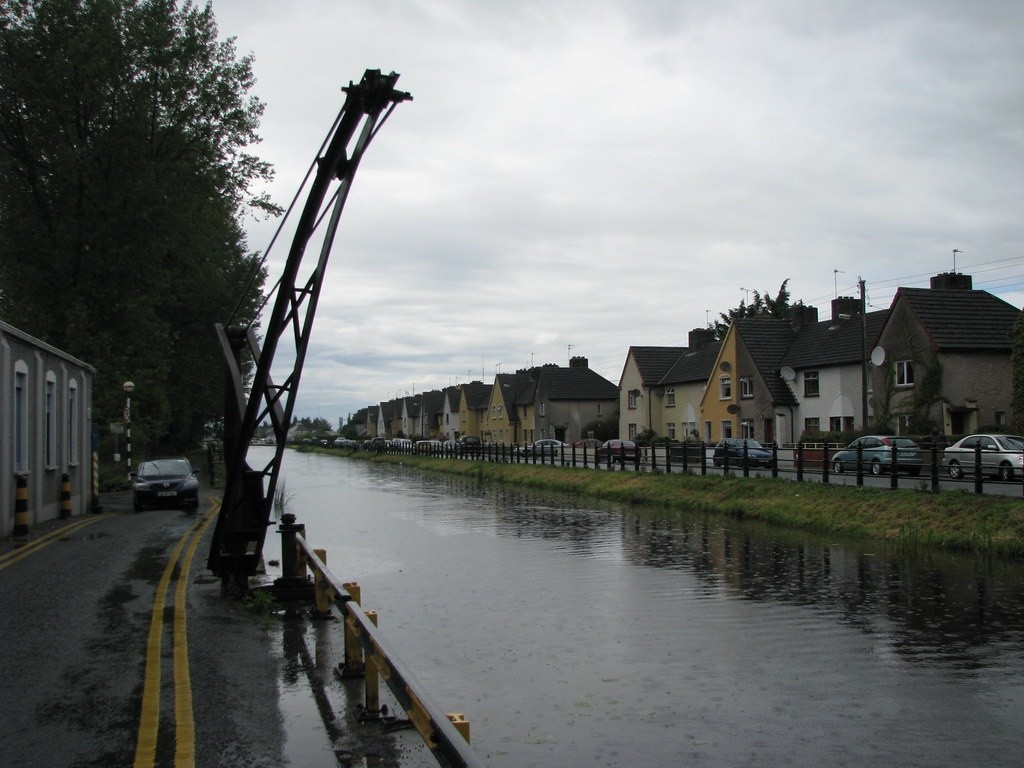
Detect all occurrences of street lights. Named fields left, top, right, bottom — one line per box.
left=836, top=312, right=871, bottom=436
left=369, top=412, right=378, bottom=437
left=413, top=397, right=424, bottom=440
left=121, top=380, right=137, bottom=492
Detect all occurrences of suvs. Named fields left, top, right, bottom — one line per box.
left=713, top=435, right=773, bottom=469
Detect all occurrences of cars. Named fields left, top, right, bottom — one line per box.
left=941, top=433, right=1024, bottom=483
left=830, top=435, right=924, bottom=477
left=302, top=432, right=482, bottom=458
left=513, top=438, right=571, bottom=458
left=575, top=438, right=604, bottom=449
left=129, top=457, right=202, bottom=512
left=594, top=439, right=642, bottom=464
